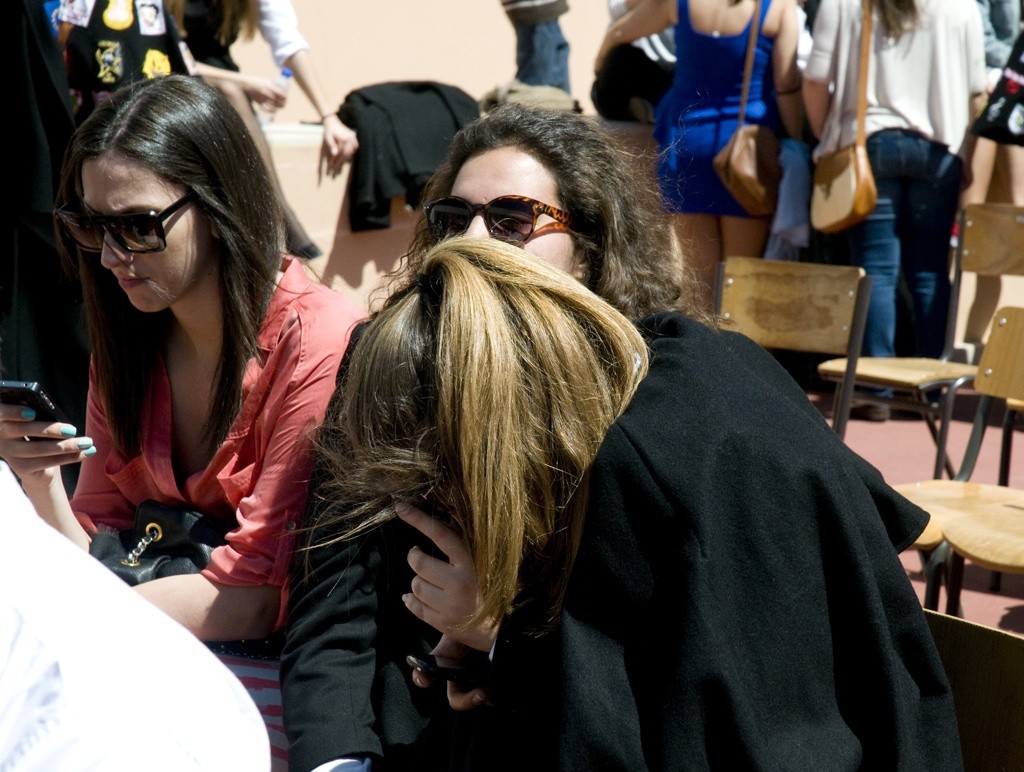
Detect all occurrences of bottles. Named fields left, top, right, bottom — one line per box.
left=258, top=67, right=293, bottom=123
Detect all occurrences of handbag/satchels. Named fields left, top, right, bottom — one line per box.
left=810, top=145, right=878, bottom=234
left=713, top=126, right=780, bottom=215
left=77, top=496, right=282, bottom=659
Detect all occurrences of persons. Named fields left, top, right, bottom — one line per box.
left=0, top=0, right=1024, bottom=772
left=319, top=234, right=968, bottom=772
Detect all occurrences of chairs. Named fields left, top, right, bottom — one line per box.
left=598, top=204, right=1024, bottom=772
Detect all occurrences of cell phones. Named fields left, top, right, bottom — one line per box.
left=0, top=380, right=68, bottom=441
left=407, top=656, right=494, bottom=691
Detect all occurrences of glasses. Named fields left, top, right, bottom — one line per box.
left=422, top=197, right=575, bottom=249
left=55, top=189, right=196, bottom=253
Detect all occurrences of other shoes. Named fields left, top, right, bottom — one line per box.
left=887, top=391, right=944, bottom=418
left=813, top=398, right=890, bottom=422
